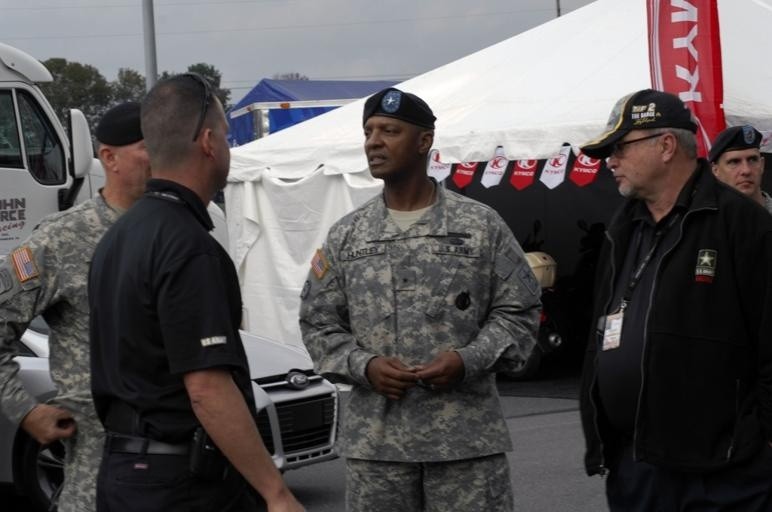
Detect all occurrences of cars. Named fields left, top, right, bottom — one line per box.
left=1, top=309, right=341, bottom=508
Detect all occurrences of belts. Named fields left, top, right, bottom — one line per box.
left=104, top=432, right=193, bottom=454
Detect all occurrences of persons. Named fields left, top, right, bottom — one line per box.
left=0, top=102, right=150, bottom=512
left=581, top=89, right=771, bottom=512
left=88, top=75, right=308, bottom=512
left=298, top=89, right=542, bottom=512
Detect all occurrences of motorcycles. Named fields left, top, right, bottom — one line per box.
left=520, top=219, right=602, bottom=253
left=496, top=252, right=564, bottom=379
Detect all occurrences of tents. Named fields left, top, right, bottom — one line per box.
left=221, top=1, right=771, bottom=352
left=228, top=77, right=402, bottom=147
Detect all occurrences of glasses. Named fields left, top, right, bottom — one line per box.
left=182, top=73, right=212, bottom=141
left=612, top=133, right=663, bottom=157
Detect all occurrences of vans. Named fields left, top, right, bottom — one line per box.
left=0, top=43, right=108, bottom=266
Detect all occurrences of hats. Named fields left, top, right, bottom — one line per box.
left=578, top=89, right=698, bottom=160
left=364, top=88, right=437, bottom=130
left=95, top=102, right=144, bottom=146
left=709, top=126, right=763, bottom=163
